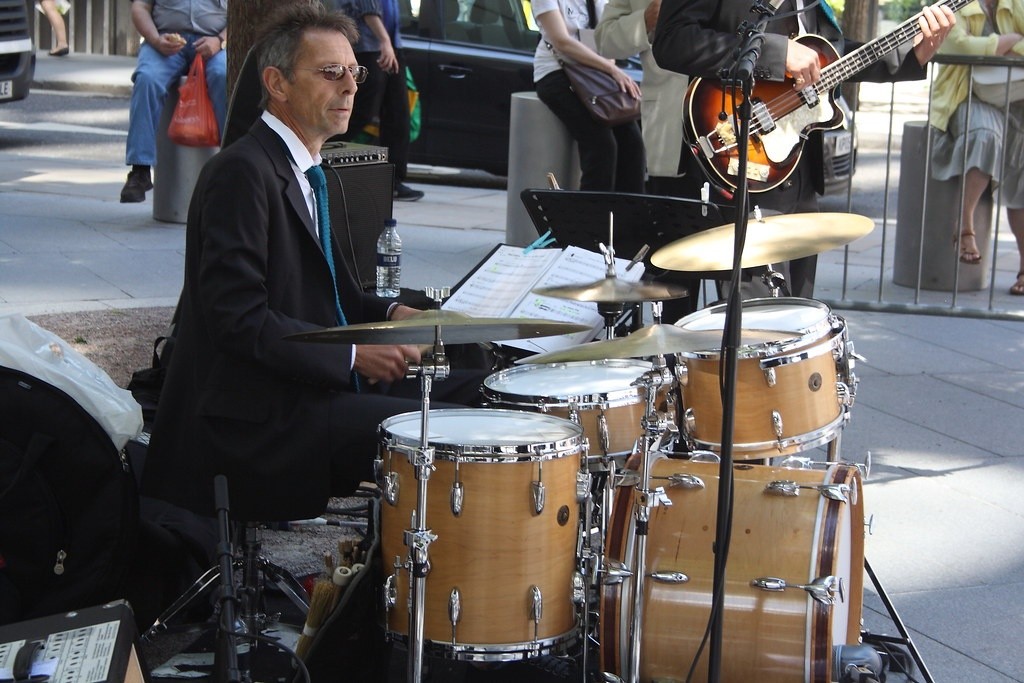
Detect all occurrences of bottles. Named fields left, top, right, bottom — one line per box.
left=216, top=600, right=251, bottom=678
left=377, top=219, right=402, bottom=298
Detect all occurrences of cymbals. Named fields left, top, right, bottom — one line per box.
left=529, top=278, right=690, bottom=303
left=281, top=309, right=595, bottom=345
left=513, top=323, right=807, bottom=364
left=650, top=212, right=875, bottom=272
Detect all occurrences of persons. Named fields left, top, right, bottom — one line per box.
left=119, top=0, right=228, bottom=206
left=35, top=0, right=71, bottom=56
left=928, top=0, right=1024, bottom=296
left=528, top=1, right=955, bottom=469
left=332, top=0, right=423, bottom=201
left=151, top=6, right=489, bottom=683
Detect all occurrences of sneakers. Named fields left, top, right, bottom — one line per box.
left=120, top=170, right=154, bottom=203
left=393, top=183, right=424, bottom=202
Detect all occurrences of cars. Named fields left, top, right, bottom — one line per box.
left=354, top=1, right=858, bottom=199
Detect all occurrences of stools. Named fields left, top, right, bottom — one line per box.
left=139, top=489, right=312, bottom=683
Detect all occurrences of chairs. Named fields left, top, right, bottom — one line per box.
left=444, top=0, right=471, bottom=43
left=470, top=0, right=513, bottom=49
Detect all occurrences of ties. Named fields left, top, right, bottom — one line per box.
left=305, top=165, right=361, bottom=393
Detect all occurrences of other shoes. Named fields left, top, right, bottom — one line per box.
left=48, top=47, right=68, bottom=57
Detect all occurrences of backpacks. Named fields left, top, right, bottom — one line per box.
left=0, top=326, right=178, bottom=629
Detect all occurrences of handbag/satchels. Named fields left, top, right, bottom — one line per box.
left=559, top=57, right=642, bottom=126
left=167, top=53, right=221, bottom=147
left=970, top=58, right=1024, bottom=107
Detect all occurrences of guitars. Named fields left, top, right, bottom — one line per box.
left=681, top=0, right=975, bottom=193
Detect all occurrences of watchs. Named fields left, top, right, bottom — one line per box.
left=215, top=35, right=226, bottom=50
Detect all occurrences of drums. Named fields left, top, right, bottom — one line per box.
left=379, top=408, right=583, bottom=663
left=674, top=296, right=847, bottom=460
left=478, top=359, right=671, bottom=464
left=599, top=451, right=865, bottom=683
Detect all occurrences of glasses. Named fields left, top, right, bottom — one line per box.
left=288, top=65, right=368, bottom=84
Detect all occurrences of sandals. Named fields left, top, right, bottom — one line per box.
left=952, top=230, right=984, bottom=265
left=1009, top=271, right=1024, bottom=295
